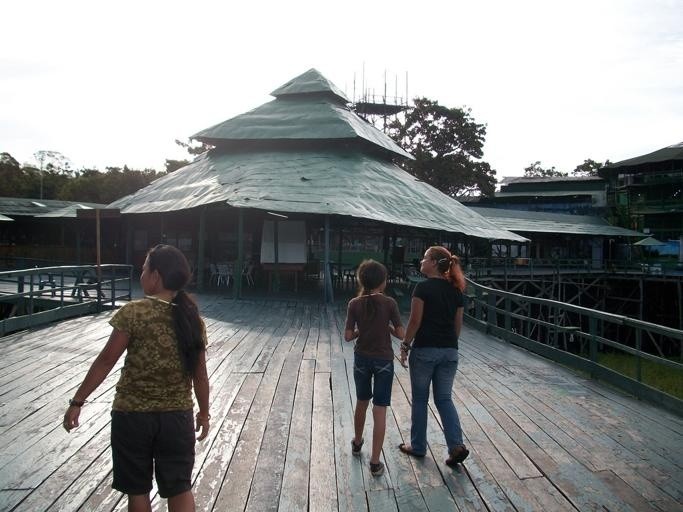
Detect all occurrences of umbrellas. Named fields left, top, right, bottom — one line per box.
left=631, top=237, right=668, bottom=258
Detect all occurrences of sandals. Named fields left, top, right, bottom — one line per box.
left=399, top=443, right=426, bottom=457
left=446, top=444, right=469, bottom=465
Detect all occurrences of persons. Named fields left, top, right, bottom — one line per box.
left=60, top=244, right=212, bottom=511
left=341, top=258, right=404, bottom=477
left=397, top=245, right=469, bottom=466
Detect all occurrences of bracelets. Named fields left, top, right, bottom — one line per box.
left=68, top=397, right=87, bottom=407
left=195, top=412, right=210, bottom=422
left=399, top=341, right=411, bottom=356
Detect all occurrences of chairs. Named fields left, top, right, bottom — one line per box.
left=384, top=262, right=402, bottom=284
left=330, top=263, right=361, bottom=287
left=210, top=259, right=255, bottom=286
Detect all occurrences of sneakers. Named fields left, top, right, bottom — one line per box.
left=351, top=438, right=364, bottom=455
left=370, top=462, right=384, bottom=476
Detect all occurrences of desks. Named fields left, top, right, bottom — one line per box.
left=404, top=262, right=428, bottom=289
